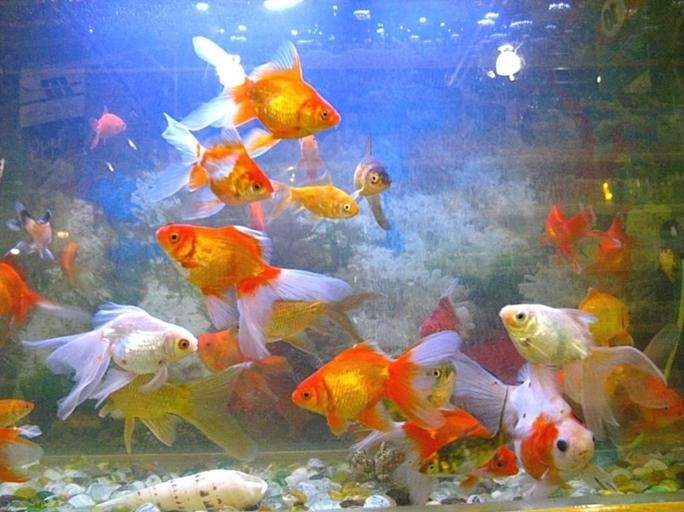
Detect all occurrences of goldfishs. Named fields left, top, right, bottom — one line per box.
left=0, top=35, right=684, bottom=511
